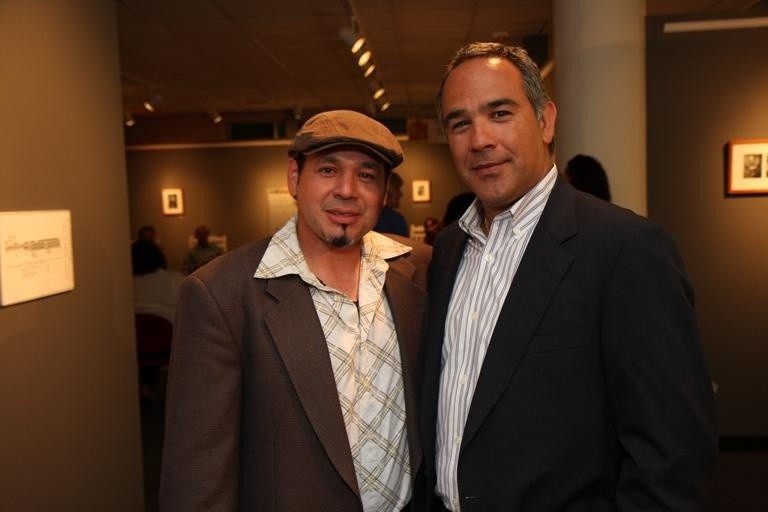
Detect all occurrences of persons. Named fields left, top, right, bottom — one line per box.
left=152, top=109, right=434, bottom=511
left=179, top=224, right=225, bottom=273
left=422, top=217, right=443, bottom=245
left=373, top=170, right=408, bottom=238
left=414, top=42, right=717, bottom=511
left=131, top=225, right=168, bottom=277
left=564, top=154, right=611, bottom=202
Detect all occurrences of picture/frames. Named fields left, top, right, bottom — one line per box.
left=725, top=135, right=768, bottom=197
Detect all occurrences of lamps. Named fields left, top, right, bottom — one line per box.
left=337, top=22, right=390, bottom=116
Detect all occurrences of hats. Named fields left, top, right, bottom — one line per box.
left=288, top=109, right=405, bottom=171
